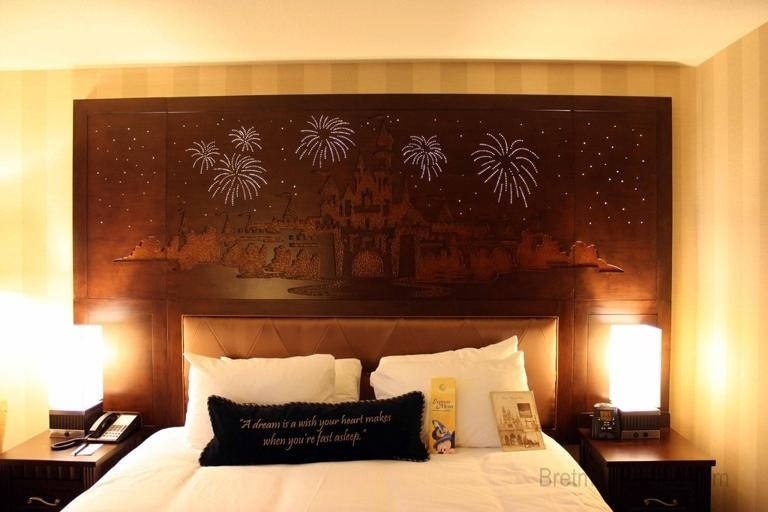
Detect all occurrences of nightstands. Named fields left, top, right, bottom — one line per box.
left=2, top=428, right=137, bottom=512
left=581, top=427, right=716, bottom=512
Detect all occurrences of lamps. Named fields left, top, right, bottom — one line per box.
left=48, top=325, right=105, bottom=431
left=611, top=325, right=664, bottom=441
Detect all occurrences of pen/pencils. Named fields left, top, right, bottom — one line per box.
left=74, top=443, right=90, bottom=456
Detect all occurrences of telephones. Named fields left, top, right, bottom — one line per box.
left=50, top=412, right=142, bottom=451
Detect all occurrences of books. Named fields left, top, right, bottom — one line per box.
left=490, top=390, right=545, bottom=452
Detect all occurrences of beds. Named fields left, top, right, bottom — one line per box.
left=57, top=313, right=612, bottom=511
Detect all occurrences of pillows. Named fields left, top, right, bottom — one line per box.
left=178, top=334, right=532, bottom=465
